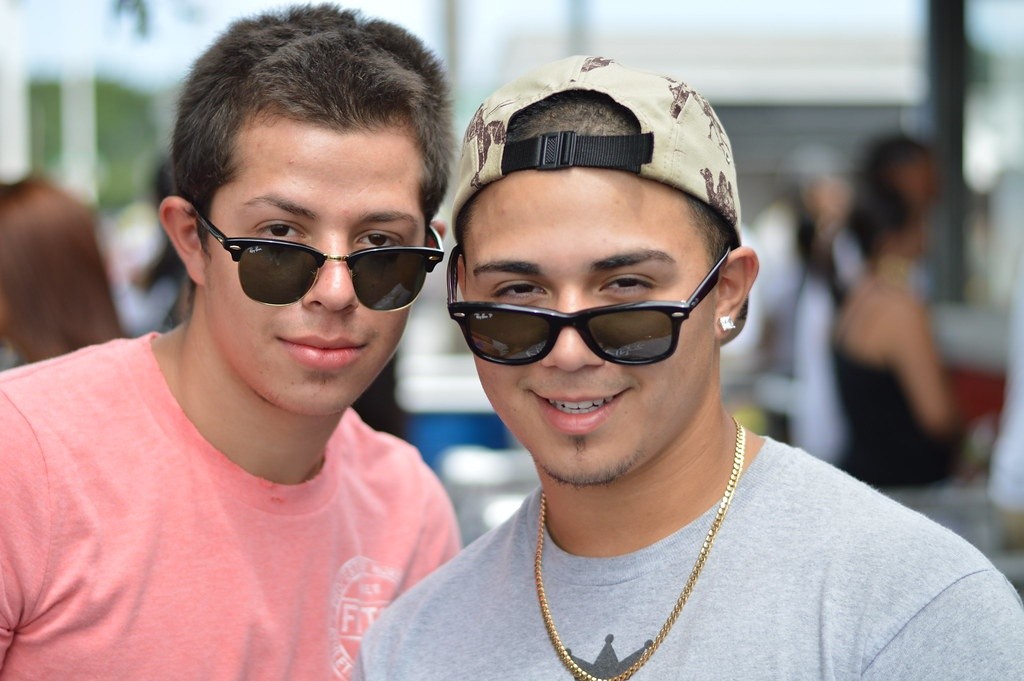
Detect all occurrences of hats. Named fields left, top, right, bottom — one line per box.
left=450, top=55, right=749, bottom=346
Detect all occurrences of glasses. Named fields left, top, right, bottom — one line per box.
left=446, top=243, right=732, bottom=364
left=193, top=209, right=444, bottom=311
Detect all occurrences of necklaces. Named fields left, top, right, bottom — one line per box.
left=534, top=416, right=746, bottom=681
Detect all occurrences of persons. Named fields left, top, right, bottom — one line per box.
left=0, top=180, right=124, bottom=364
left=0, top=3, right=461, bottom=681
left=749, top=135, right=1023, bottom=552
left=354, top=57, right=1024, bottom=681
